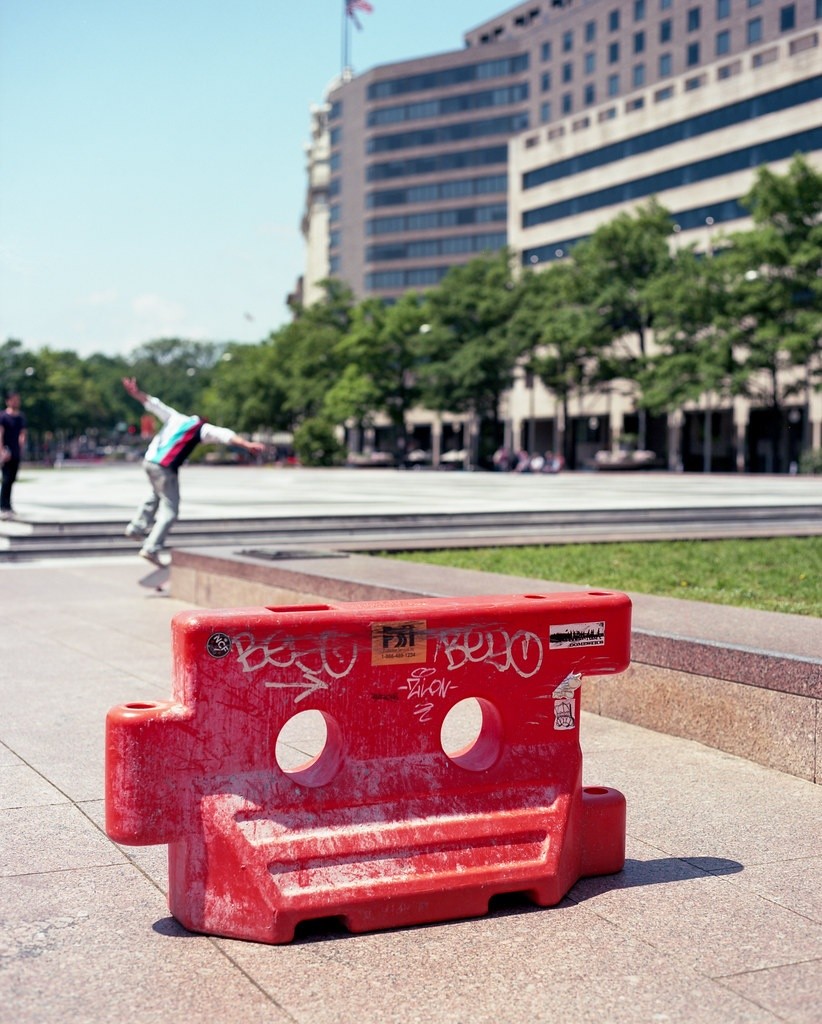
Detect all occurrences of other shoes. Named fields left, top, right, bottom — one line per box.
left=126, top=528, right=147, bottom=540
left=138, top=549, right=167, bottom=569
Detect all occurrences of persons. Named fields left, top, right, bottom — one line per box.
left=0, top=389, right=29, bottom=519
left=120, top=375, right=269, bottom=565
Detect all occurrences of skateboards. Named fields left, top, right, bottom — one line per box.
left=137, top=562, right=172, bottom=593
